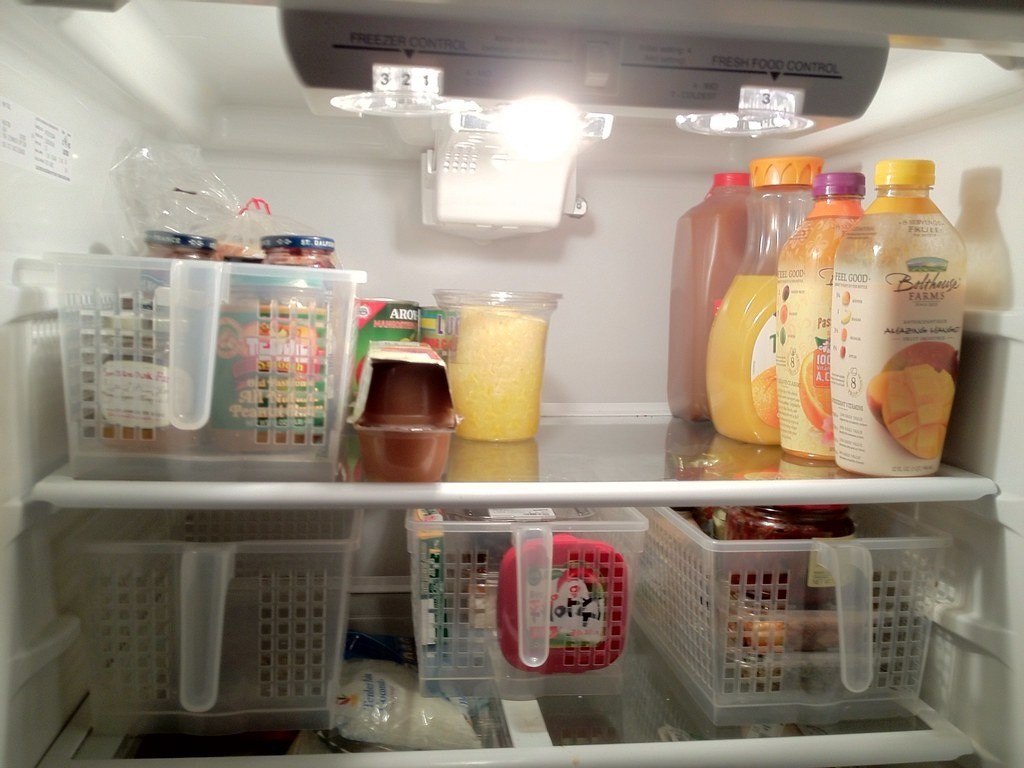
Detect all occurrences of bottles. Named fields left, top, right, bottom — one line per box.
left=829, top=158, right=969, bottom=479
left=666, top=170, right=751, bottom=422
left=775, top=172, right=867, bottom=462
left=705, top=156, right=826, bottom=446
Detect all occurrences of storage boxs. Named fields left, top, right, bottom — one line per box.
left=50, top=253, right=367, bottom=488
left=71, top=506, right=956, bottom=728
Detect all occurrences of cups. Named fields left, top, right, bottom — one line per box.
left=432, top=288, right=563, bottom=443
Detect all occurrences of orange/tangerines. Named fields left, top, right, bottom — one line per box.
left=751, top=366, right=779, bottom=428
left=798, top=352, right=832, bottom=427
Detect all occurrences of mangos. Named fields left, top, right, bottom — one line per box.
left=878, top=364, right=955, bottom=459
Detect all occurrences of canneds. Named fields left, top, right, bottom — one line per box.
left=139, top=228, right=461, bottom=440
left=694, top=504, right=859, bottom=613
